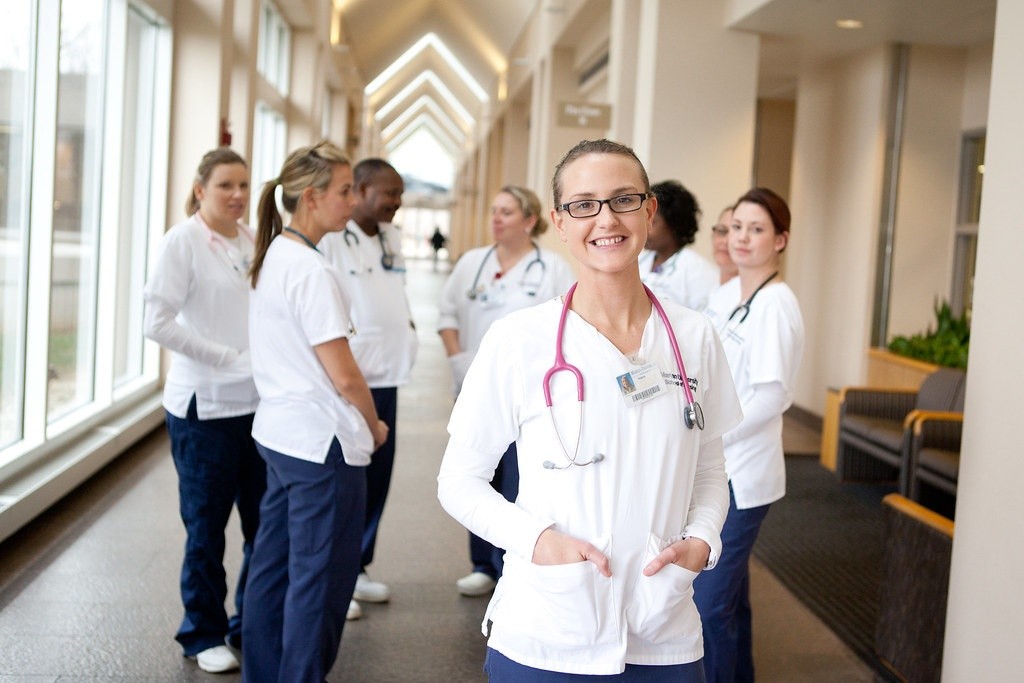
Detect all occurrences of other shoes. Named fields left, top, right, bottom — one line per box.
left=346, top=598, right=361, bottom=621
left=186, top=644, right=239, bottom=673
left=352, top=571, right=390, bottom=602
left=456, top=571, right=497, bottom=596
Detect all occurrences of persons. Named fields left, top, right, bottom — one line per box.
left=142, top=149, right=258, bottom=672
left=437, top=185, right=577, bottom=596
left=437, top=139, right=743, bottom=683
left=242, top=138, right=416, bottom=683
left=638, top=180, right=803, bottom=683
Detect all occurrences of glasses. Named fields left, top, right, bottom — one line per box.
left=558, top=192, right=649, bottom=218
left=713, top=225, right=729, bottom=236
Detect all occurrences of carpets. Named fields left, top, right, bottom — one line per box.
left=749, top=453, right=956, bottom=683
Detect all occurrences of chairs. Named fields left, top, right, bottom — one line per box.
left=817, top=365, right=968, bottom=508
left=883, top=410, right=964, bottom=549
